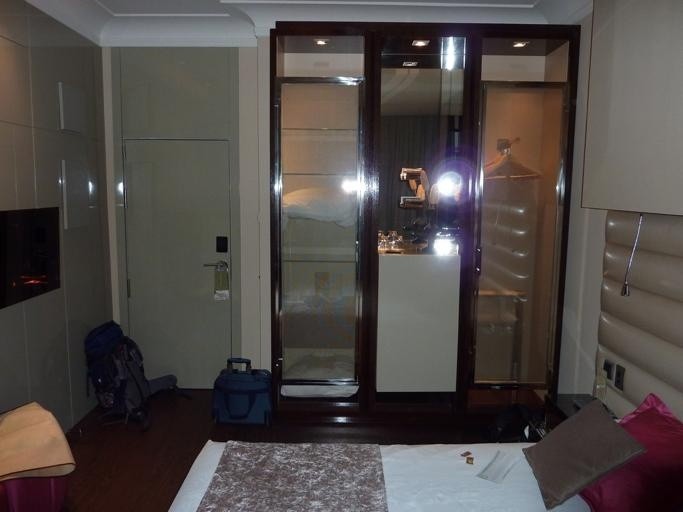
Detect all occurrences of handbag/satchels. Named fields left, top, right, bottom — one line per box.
left=213, top=359, right=272, bottom=428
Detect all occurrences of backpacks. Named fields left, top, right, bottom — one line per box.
left=84, top=320, right=175, bottom=429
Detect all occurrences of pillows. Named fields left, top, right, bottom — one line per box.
left=522, top=393, right=681, bottom=511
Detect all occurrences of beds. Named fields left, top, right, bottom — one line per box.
left=167, top=438, right=591, bottom=511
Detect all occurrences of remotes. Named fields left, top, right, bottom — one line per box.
left=573, top=399, right=587, bottom=409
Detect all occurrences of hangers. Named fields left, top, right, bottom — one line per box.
left=483, top=145, right=543, bottom=181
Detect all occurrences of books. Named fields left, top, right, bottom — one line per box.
left=572, top=398, right=593, bottom=411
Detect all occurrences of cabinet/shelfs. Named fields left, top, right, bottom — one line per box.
left=268, top=22, right=581, bottom=428
